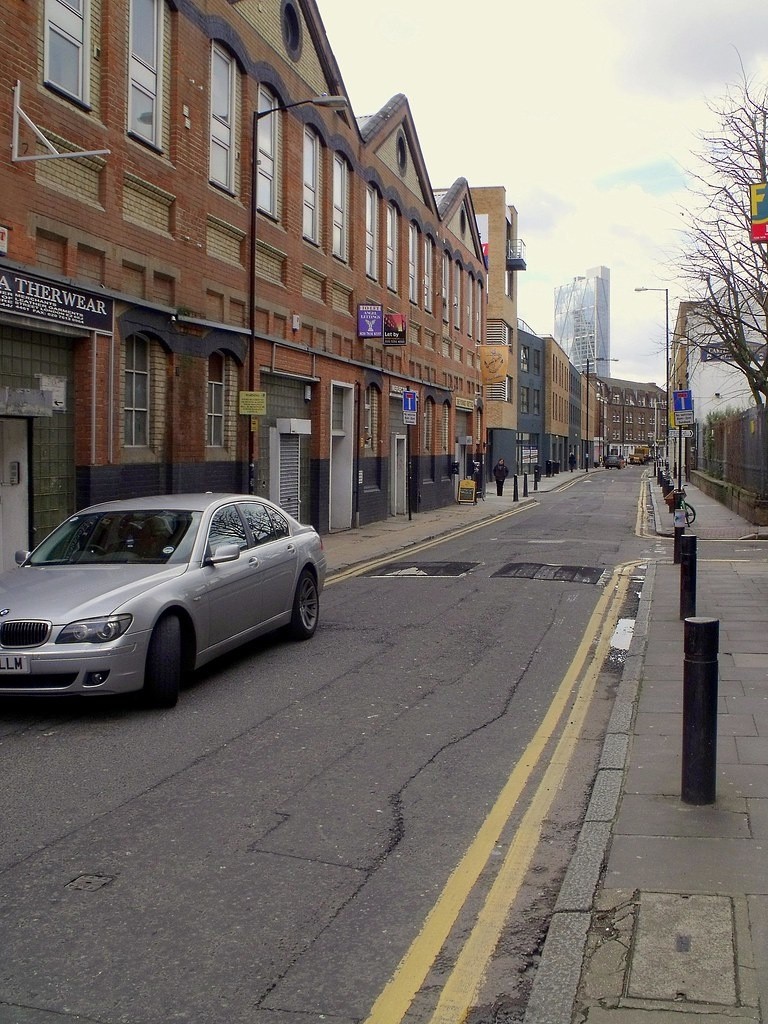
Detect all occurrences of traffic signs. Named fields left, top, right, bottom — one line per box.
left=668, top=430, right=694, bottom=437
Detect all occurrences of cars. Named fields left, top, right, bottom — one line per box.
left=0, top=492, right=326, bottom=709
left=605, top=454, right=623, bottom=470
left=633, top=459, right=641, bottom=466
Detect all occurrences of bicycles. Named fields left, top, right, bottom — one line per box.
left=674, top=483, right=699, bottom=526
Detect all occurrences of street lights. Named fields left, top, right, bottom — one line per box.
left=635, top=287, right=670, bottom=469
left=586, top=357, right=604, bottom=474
left=247, top=93, right=350, bottom=495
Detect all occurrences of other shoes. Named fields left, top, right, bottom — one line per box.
left=571, top=470, right=572, bottom=472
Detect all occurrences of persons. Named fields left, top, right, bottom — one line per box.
left=569, top=454, right=575, bottom=472
left=599, top=455, right=603, bottom=468
left=493, top=459, right=509, bottom=496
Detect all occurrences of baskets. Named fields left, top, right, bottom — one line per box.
left=665, top=488, right=687, bottom=505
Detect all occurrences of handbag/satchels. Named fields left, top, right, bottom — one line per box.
left=574, top=460, right=576, bottom=464
